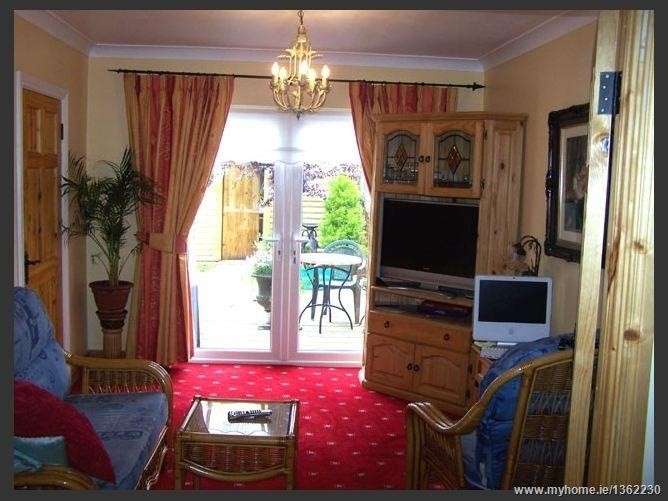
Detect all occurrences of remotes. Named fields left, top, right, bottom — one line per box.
left=229, top=409, right=273, bottom=421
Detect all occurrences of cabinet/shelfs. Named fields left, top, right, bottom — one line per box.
left=361, top=111, right=529, bottom=416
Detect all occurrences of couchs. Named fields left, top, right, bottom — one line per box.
left=14, top=287, right=173, bottom=490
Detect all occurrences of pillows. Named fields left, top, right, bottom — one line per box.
left=14, top=379, right=117, bottom=484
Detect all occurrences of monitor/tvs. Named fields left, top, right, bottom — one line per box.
left=472, top=275, right=553, bottom=345
left=377, top=194, right=481, bottom=300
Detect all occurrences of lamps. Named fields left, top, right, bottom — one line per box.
left=269, top=9, right=333, bottom=120
left=513, top=235, right=542, bottom=276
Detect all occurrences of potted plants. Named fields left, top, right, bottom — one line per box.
left=252, top=264, right=272, bottom=330
left=58, top=145, right=167, bottom=312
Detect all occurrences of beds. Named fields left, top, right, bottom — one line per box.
left=298, top=252, right=362, bottom=334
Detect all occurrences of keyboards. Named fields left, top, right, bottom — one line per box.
left=479, top=346, right=509, bottom=360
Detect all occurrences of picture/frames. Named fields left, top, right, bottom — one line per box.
left=543, top=102, right=605, bottom=269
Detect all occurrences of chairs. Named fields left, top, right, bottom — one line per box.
left=311, top=240, right=366, bottom=324
left=407, top=328, right=600, bottom=490
